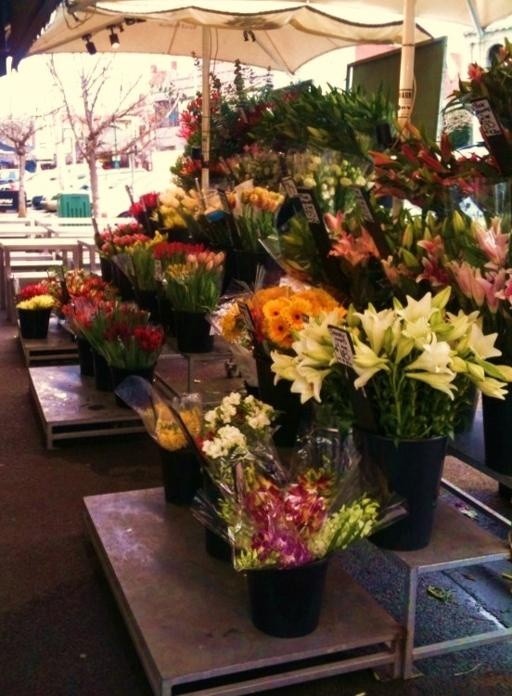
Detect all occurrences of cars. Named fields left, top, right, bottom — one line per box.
left=0, top=157, right=169, bottom=217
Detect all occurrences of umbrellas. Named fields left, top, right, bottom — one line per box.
left=22, top=7, right=433, bottom=192
left=275, top=1, right=512, bottom=133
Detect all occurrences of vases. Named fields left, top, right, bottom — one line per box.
left=248, top=555, right=330, bottom=638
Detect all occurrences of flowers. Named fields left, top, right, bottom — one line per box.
left=14, top=35, right=512, bottom=574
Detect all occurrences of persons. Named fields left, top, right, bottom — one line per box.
left=8, top=180, right=16, bottom=191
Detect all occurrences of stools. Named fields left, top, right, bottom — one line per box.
left=0, top=237, right=82, bottom=320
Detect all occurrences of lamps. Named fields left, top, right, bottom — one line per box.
left=107, top=25, right=120, bottom=47
